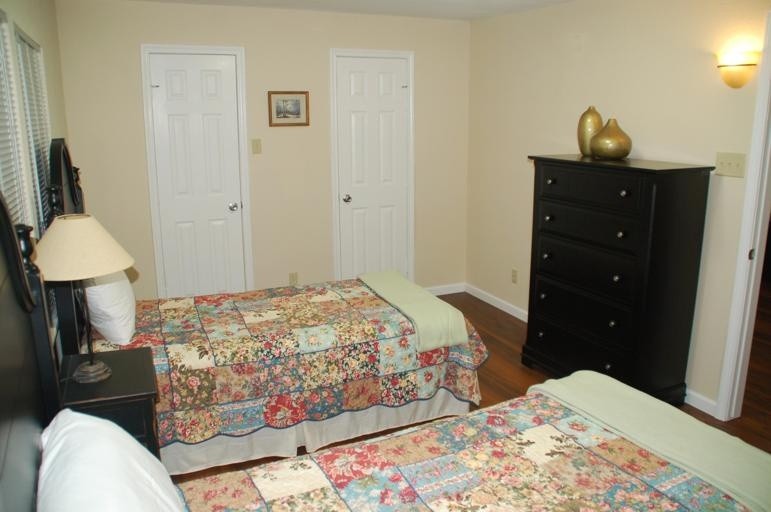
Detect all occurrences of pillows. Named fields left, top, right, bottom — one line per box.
left=84, top=276, right=140, bottom=346
left=35, top=403, right=188, bottom=512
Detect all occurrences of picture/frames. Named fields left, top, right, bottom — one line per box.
left=267, top=91, right=310, bottom=128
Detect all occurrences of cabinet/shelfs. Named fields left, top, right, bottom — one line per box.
left=522, top=155, right=714, bottom=408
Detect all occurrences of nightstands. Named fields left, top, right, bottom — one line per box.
left=60, top=346, right=158, bottom=462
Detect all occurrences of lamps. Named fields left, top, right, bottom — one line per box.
left=31, top=210, right=135, bottom=383
left=718, top=63, right=760, bottom=89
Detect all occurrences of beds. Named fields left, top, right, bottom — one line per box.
left=78, top=268, right=478, bottom=456
left=47, top=363, right=771, bottom=512
